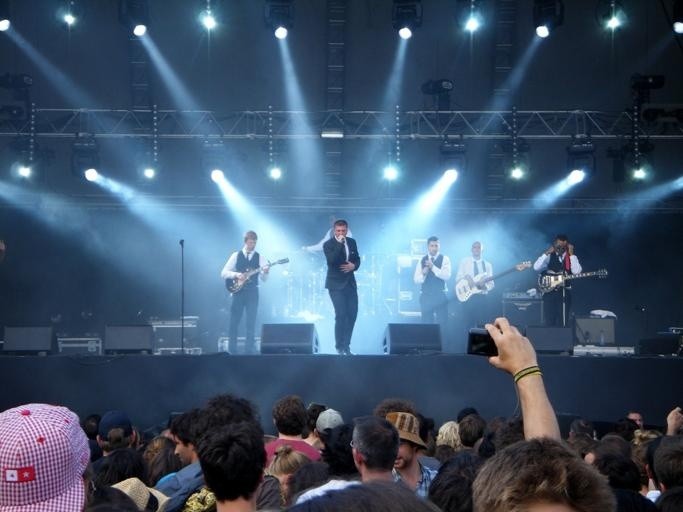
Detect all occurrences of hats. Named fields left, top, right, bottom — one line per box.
left=384, top=412, right=429, bottom=450
left=315, top=408, right=344, bottom=436
left=97, top=410, right=132, bottom=441
left=0, top=403, right=92, bottom=512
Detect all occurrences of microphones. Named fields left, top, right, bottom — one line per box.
left=180, top=239, right=184, bottom=249
left=342, top=238, right=345, bottom=244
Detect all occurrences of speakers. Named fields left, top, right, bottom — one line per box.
left=104, top=323, right=154, bottom=354
left=524, top=325, right=573, bottom=355
left=3, top=326, right=59, bottom=356
left=382, top=324, right=441, bottom=354
left=260, top=324, right=319, bottom=353
left=634, top=333, right=680, bottom=356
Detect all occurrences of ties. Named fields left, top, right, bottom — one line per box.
left=343, top=240, right=346, bottom=262
left=246, top=252, right=250, bottom=263
left=473, top=261, right=478, bottom=280
left=430, top=256, right=435, bottom=264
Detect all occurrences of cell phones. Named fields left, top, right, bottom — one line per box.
left=466, top=328, right=503, bottom=356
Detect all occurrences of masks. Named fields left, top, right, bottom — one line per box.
left=553, top=245, right=567, bottom=255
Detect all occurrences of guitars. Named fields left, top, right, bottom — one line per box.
left=225, top=258, right=290, bottom=296
left=454, top=260, right=534, bottom=303
left=537, top=268, right=609, bottom=296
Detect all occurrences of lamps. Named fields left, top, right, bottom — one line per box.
left=392, top=1, right=423, bottom=39
left=71, top=140, right=102, bottom=181
left=533, top=1, right=565, bottom=38
left=567, top=141, right=598, bottom=180
left=421, top=77, right=455, bottom=113
left=439, top=142, right=469, bottom=178
left=200, top=148, right=226, bottom=183
left=265, top=2, right=293, bottom=39
left=119, top=2, right=147, bottom=37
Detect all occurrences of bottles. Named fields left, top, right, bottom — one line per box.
left=600, top=329, right=606, bottom=345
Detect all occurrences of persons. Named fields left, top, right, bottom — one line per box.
left=533, top=237, right=583, bottom=326
left=302, top=211, right=352, bottom=254
left=455, top=242, right=494, bottom=333
left=1, top=316, right=682, bottom=511
left=219, top=232, right=269, bottom=354
left=322, top=220, right=360, bottom=356
left=414, top=236, right=451, bottom=325
